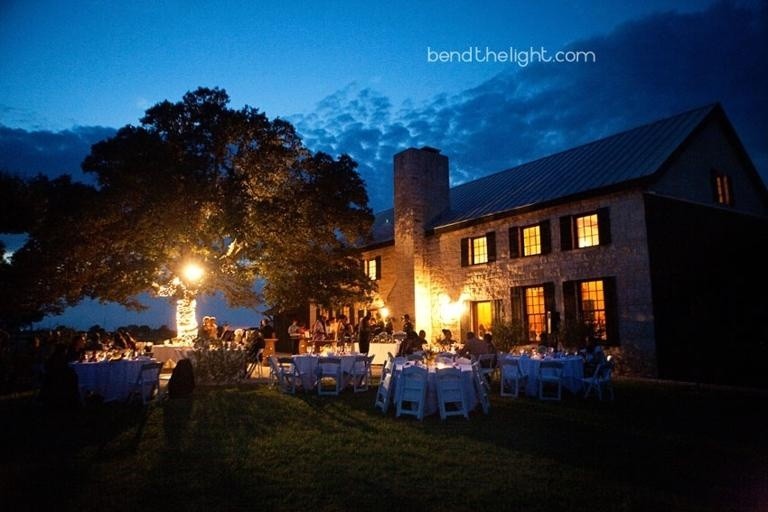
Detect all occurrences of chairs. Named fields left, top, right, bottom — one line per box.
left=45, top=337, right=615, bottom=421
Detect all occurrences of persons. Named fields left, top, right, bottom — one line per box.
left=484, top=335, right=497, bottom=369
left=286, top=309, right=428, bottom=368
left=459, top=331, right=492, bottom=386
left=441, top=328, right=458, bottom=343
left=23, top=317, right=277, bottom=374
left=581, top=333, right=606, bottom=378
